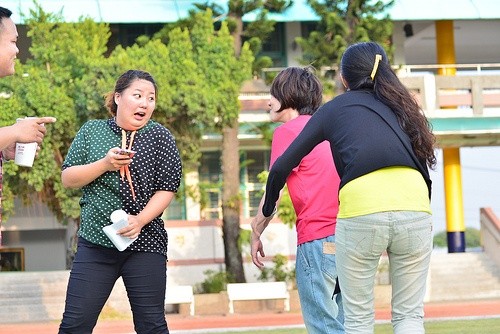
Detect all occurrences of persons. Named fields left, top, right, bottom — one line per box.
left=251, top=67, right=345, bottom=334
left=251, top=42, right=433, bottom=334
left=0, top=6, right=56, bottom=247
left=58, top=70, right=183, bottom=334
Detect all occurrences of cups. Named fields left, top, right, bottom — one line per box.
left=15, top=117, right=45, bottom=168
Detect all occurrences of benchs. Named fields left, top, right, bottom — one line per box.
left=165, top=286, right=195, bottom=316
left=226, top=282, right=290, bottom=314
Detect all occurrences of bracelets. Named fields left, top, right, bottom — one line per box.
left=269, top=206, right=278, bottom=217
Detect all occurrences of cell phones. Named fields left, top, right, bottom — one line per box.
left=117, top=149, right=137, bottom=159
